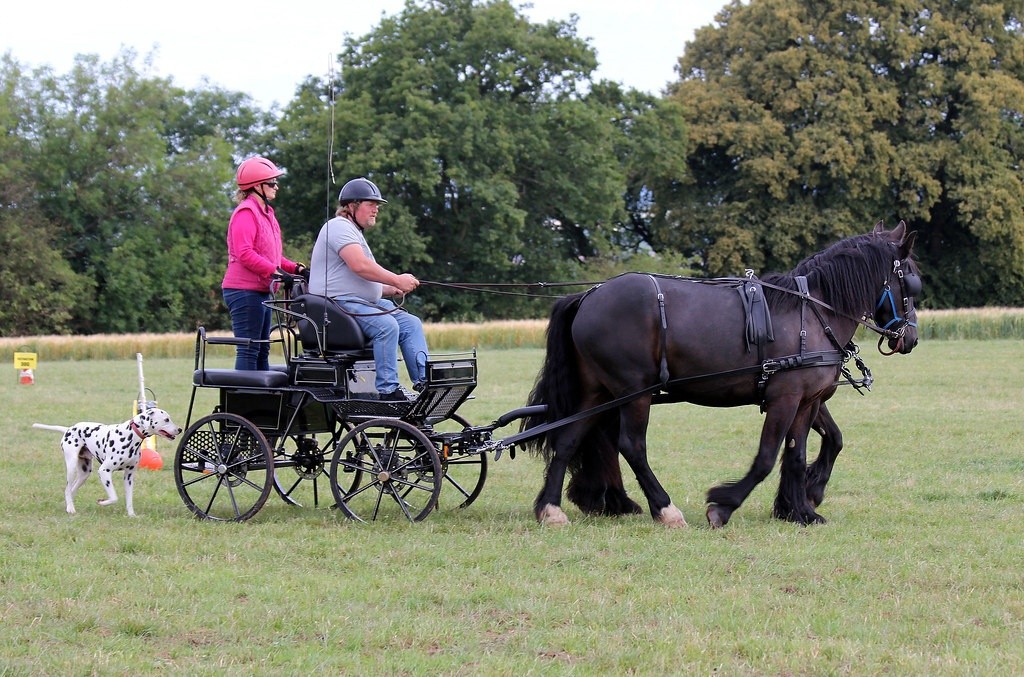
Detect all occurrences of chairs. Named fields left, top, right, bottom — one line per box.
left=289, top=291, right=375, bottom=354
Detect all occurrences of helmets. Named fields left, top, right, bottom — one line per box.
left=338, top=176, right=388, bottom=206
left=236, top=157, right=283, bottom=191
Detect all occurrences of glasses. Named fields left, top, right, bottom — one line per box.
left=259, top=180, right=278, bottom=189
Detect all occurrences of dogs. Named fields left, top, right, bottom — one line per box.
left=31, top=408, right=182, bottom=518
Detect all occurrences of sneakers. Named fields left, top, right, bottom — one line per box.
left=379, top=391, right=409, bottom=417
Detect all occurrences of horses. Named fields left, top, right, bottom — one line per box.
left=517, top=219, right=918, bottom=529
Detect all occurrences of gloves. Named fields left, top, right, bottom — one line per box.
left=271, top=264, right=293, bottom=294
left=298, top=267, right=311, bottom=283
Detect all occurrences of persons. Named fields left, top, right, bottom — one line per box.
left=220, top=156, right=310, bottom=373
left=307, top=175, right=433, bottom=418
